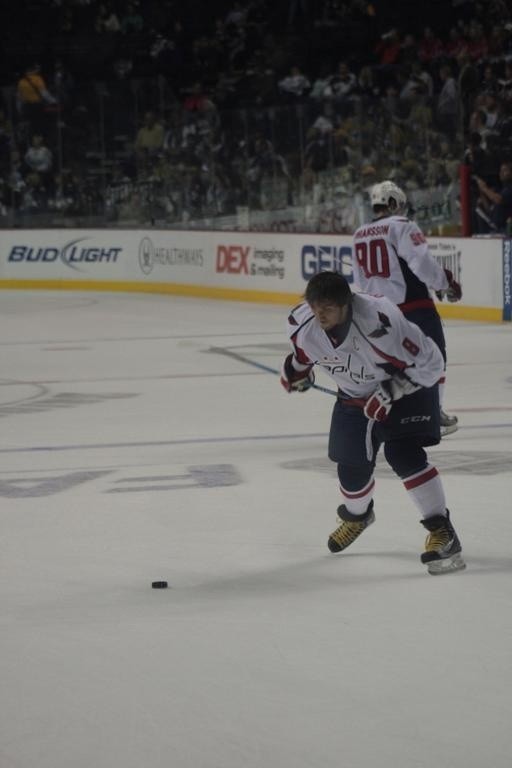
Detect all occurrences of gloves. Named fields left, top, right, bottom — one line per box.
left=282, top=354, right=314, bottom=392
left=437, top=270, right=461, bottom=302
left=363, top=379, right=395, bottom=422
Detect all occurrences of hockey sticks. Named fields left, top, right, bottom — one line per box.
left=177, top=341, right=366, bottom=408
left=315, top=244, right=453, bottom=296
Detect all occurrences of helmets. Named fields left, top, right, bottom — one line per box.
left=370, top=181, right=406, bottom=212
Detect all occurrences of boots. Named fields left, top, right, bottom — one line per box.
left=328, top=499, right=374, bottom=552
left=421, top=508, right=461, bottom=562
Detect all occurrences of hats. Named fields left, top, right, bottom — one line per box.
left=306, top=272, right=352, bottom=302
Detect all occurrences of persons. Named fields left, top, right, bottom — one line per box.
left=0, top=0, right=510, bottom=242
left=281, top=270, right=463, bottom=562
left=351, top=178, right=461, bottom=431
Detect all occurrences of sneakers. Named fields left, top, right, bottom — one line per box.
left=440, top=411, right=457, bottom=426
